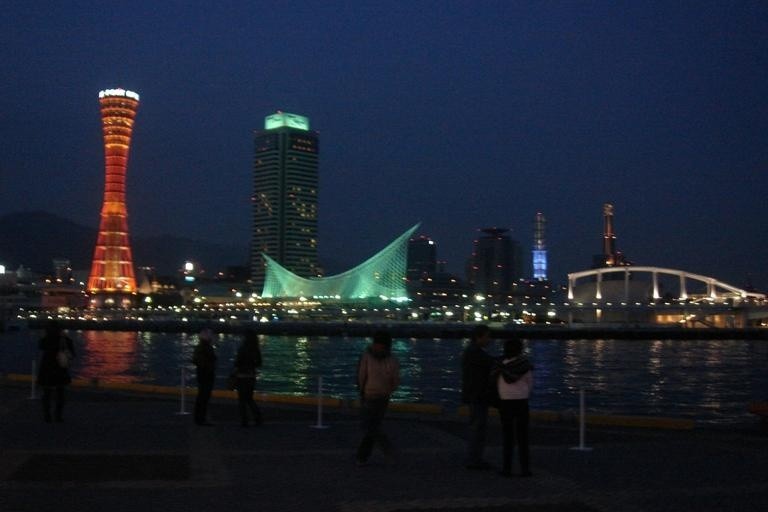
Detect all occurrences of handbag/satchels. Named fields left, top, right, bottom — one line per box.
left=55, top=338, right=71, bottom=370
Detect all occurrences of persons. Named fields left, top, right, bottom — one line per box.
left=231, top=331, right=264, bottom=428
left=497, top=339, right=536, bottom=480
left=459, top=325, right=504, bottom=470
left=353, top=330, right=401, bottom=468
left=190, top=329, right=219, bottom=427
left=35, top=324, right=76, bottom=424
